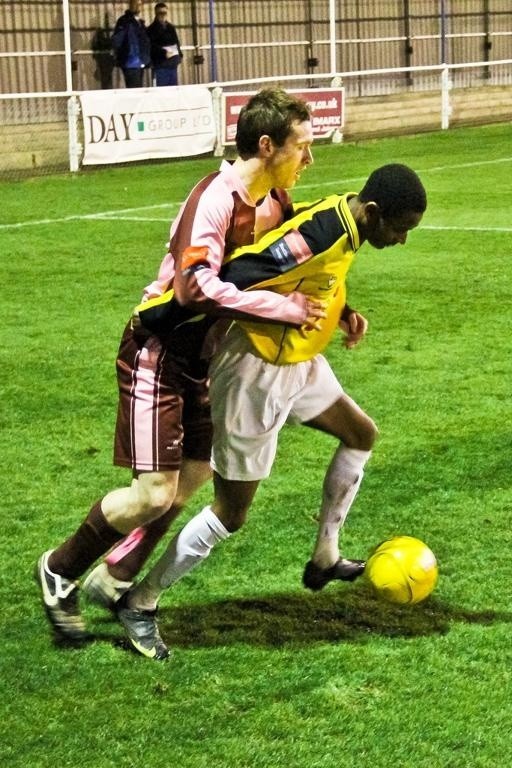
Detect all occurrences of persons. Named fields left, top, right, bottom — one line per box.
left=110, top=0, right=152, bottom=89
left=35, top=85, right=370, bottom=647
left=111, top=161, right=429, bottom=660
left=145, top=0, right=184, bottom=87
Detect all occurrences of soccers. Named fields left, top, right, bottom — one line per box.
left=364, top=535, right=438, bottom=606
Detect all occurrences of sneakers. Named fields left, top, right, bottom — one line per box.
left=302, top=558, right=367, bottom=593
left=83, top=561, right=134, bottom=613
left=111, top=592, right=169, bottom=660
left=34, top=547, right=92, bottom=646
